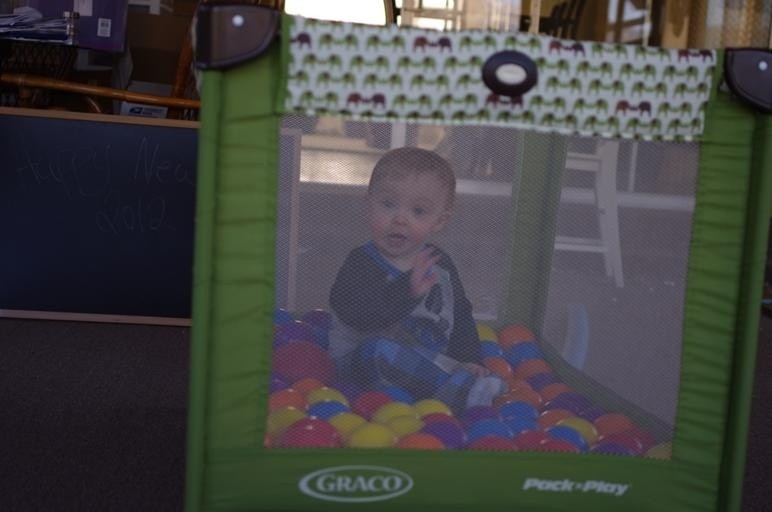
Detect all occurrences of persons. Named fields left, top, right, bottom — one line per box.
left=325, top=145, right=503, bottom=411
